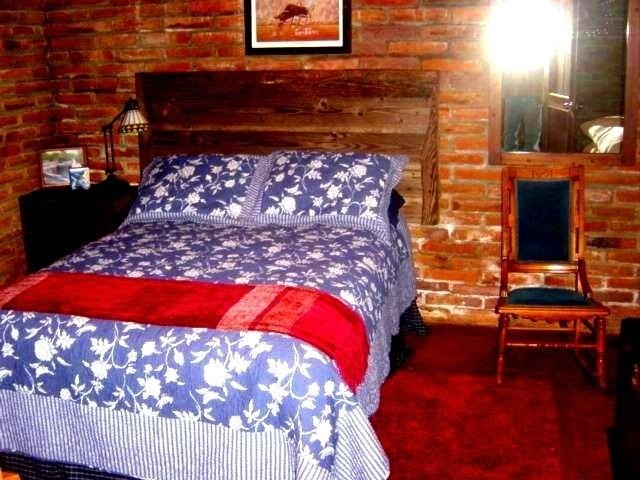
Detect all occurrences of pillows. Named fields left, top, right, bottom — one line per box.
left=117, top=150, right=411, bottom=242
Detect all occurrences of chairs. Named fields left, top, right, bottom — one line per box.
left=493, top=164, right=612, bottom=391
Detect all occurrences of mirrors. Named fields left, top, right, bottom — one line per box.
left=486, top=0, right=639, bottom=168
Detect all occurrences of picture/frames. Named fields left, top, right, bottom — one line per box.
left=243, top=1, right=353, bottom=54
left=36, top=145, right=92, bottom=190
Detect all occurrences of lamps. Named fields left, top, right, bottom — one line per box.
left=100, top=96, right=151, bottom=189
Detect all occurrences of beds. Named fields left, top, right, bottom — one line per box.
left=0, top=210, right=417, bottom=479
